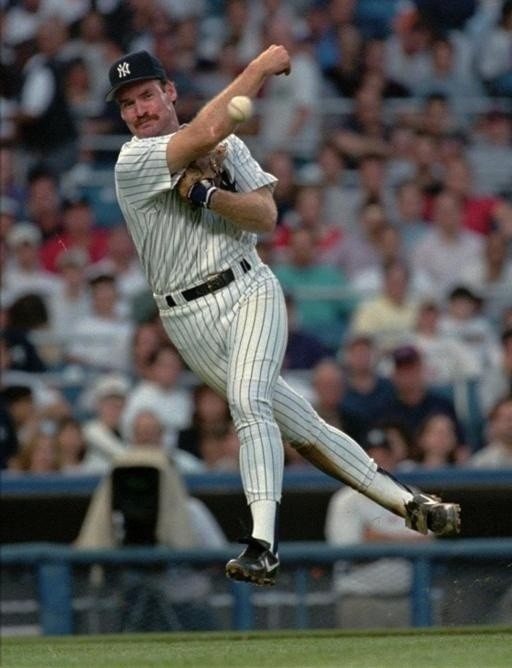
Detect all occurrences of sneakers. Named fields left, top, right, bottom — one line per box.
left=225, top=533, right=287, bottom=590
left=398, top=489, right=466, bottom=538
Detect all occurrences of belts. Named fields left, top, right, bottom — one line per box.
left=151, top=247, right=262, bottom=312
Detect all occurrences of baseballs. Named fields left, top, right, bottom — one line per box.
left=227, top=96, right=254, bottom=122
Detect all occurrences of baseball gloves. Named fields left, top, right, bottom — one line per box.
left=175, top=124, right=229, bottom=203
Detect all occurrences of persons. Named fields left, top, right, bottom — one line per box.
left=318, top=427, right=444, bottom=632
left=104, top=38, right=470, bottom=587
left=1, top=0, right=512, bottom=481
left=116, top=459, right=233, bottom=636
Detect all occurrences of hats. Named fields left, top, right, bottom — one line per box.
left=101, top=46, right=171, bottom=108
